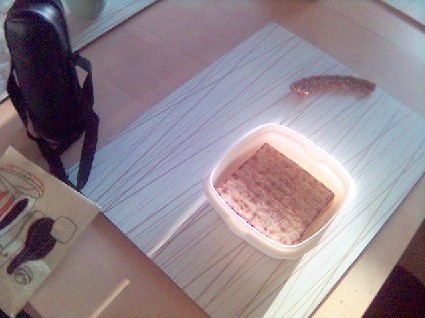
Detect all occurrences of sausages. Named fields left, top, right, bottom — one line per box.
left=290, top=73, right=376, bottom=99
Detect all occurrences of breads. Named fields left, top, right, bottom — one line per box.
left=216, top=143, right=335, bottom=245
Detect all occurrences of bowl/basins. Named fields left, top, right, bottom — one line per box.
left=204, top=123, right=357, bottom=259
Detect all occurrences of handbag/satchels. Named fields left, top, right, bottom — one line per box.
left=4, top=0, right=99, bottom=192
